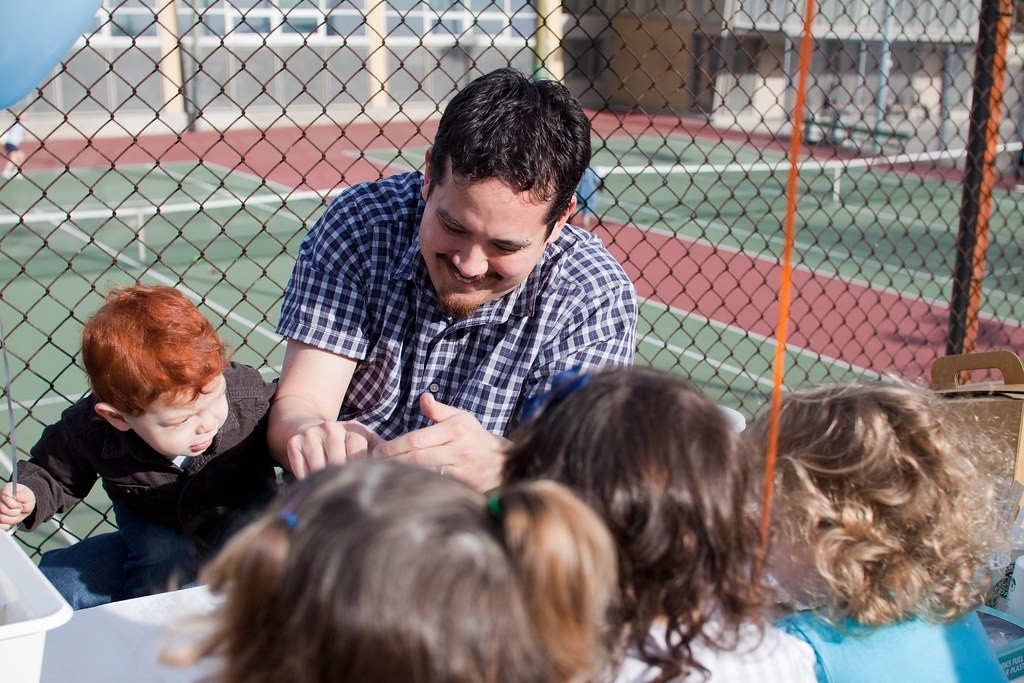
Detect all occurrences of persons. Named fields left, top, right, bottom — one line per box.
left=0, top=284, right=281, bottom=613
left=565, top=167, right=601, bottom=227
left=158, top=366, right=1024, bottom=683
left=266, top=67, right=638, bottom=494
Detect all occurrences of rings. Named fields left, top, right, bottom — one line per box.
left=436, top=465, right=444, bottom=476
